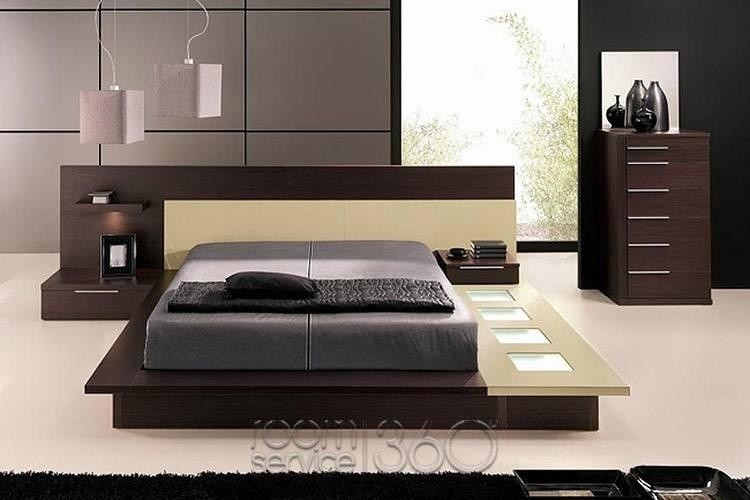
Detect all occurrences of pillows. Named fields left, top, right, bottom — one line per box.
left=224, top=271, right=320, bottom=296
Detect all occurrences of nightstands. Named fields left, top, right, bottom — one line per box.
left=435, top=249, right=520, bottom=284
left=41, top=267, right=160, bottom=320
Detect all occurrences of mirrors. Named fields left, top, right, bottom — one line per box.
left=601, top=51, right=680, bottom=134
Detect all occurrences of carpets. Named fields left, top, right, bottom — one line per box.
left=0, top=470, right=750, bottom=500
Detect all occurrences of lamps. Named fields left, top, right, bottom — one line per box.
left=79, top=0, right=145, bottom=145
left=153, top=0, right=223, bottom=119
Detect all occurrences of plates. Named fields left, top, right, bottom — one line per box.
left=447, top=253, right=471, bottom=259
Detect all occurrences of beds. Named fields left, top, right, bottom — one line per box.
left=85, top=198, right=631, bottom=430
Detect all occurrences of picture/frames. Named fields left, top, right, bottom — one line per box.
left=99, top=233, right=136, bottom=280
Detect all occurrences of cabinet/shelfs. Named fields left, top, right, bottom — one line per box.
left=599, top=130, right=713, bottom=305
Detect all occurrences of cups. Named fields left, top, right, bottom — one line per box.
left=450, top=248, right=469, bottom=255
